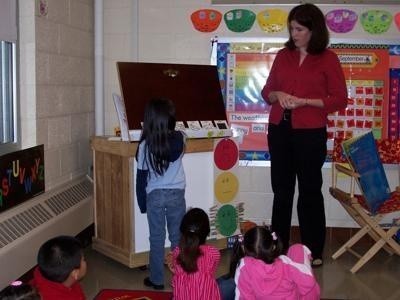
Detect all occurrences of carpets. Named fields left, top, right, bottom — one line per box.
left=92, top=287, right=174, bottom=300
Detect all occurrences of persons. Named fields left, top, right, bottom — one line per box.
left=135, top=96, right=187, bottom=290
left=27, top=236, right=87, bottom=300
left=0, top=284, right=40, bottom=300
left=230, top=226, right=321, bottom=300
left=260, top=3, right=348, bottom=270
left=167, top=208, right=222, bottom=300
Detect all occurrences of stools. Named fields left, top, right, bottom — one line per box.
left=329, top=161, right=400, bottom=274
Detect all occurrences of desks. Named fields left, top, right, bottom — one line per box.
left=89, top=134, right=246, bottom=269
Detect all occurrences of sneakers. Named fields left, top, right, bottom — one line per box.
left=144, top=277, right=165, bottom=290
